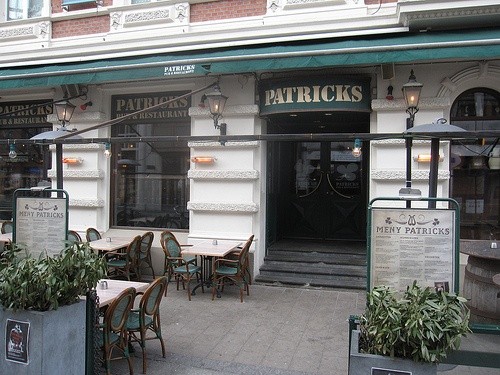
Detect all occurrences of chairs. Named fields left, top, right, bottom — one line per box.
left=0, top=220, right=255, bottom=375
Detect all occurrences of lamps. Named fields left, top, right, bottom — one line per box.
left=53, top=89, right=78, bottom=133
left=400, top=68, right=424, bottom=122
left=204, top=84, right=229, bottom=130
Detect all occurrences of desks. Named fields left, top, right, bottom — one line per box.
left=180, top=242, right=243, bottom=298
left=77, top=279, right=150, bottom=310
left=0, top=232, right=12, bottom=243
left=71, top=237, right=130, bottom=274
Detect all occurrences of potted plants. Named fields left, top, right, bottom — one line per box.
left=348, top=279, right=475, bottom=375
left=0, top=240, right=108, bottom=375
